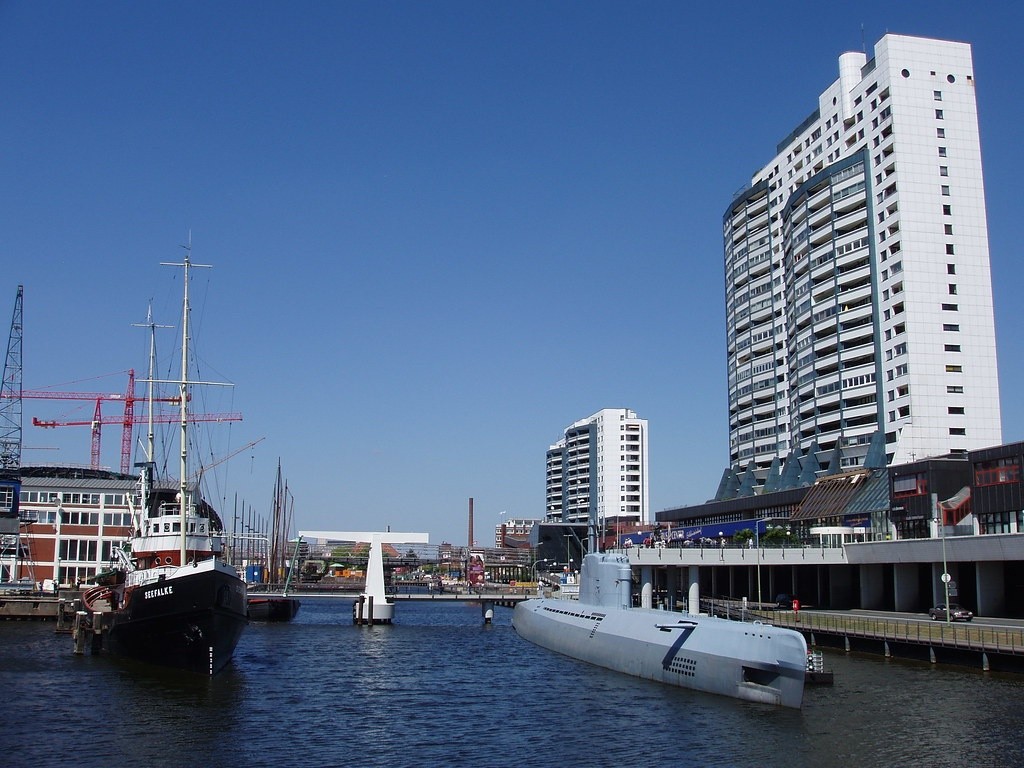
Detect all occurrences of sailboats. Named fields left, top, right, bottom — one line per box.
left=80, top=229, right=250, bottom=681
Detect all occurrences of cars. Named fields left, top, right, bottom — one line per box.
left=775, top=595, right=802, bottom=608
left=929, top=602, right=974, bottom=623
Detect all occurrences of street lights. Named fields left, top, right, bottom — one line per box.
left=531, top=558, right=547, bottom=590
left=756, top=517, right=773, bottom=610
left=581, top=537, right=589, bottom=562
left=563, top=534, right=574, bottom=571
left=548, top=562, right=557, bottom=576
left=940, top=496, right=959, bottom=627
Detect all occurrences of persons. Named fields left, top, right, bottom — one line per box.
left=468, top=580, right=472, bottom=595
left=38, top=582, right=43, bottom=597
left=76, top=575, right=81, bottom=591
left=624, top=521, right=809, bottom=549
left=52, top=580, right=58, bottom=593
left=437, top=577, right=443, bottom=596
left=469, top=555, right=484, bottom=571
left=390, top=578, right=397, bottom=598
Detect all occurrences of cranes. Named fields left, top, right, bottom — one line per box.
left=0, top=367, right=193, bottom=476
left=32, top=396, right=245, bottom=472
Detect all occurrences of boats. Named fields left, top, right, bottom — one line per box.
left=511, top=544, right=808, bottom=710
left=246, top=597, right=301, bottom=623
left=297, top=530, right=431, bottom=628
